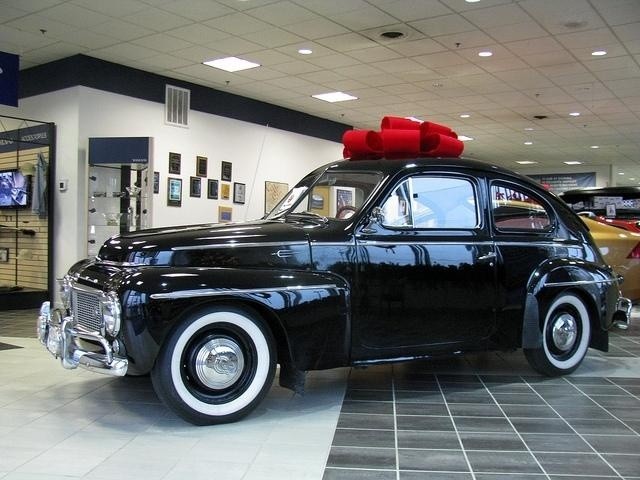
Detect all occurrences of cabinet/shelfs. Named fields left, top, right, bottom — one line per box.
left=88, top=137, right=152, bottom=259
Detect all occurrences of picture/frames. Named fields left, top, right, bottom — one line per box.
left=264, top=181, right=289, bottom=216
left=336, top=189, right=354, bottom=218
left=308, top=185, right=331, bottom=217
left=153, top=151, right=247, bottom=223
left=606, top=203, right=616, bottom=218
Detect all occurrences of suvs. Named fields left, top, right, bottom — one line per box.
left=555, top=186, right=640, bottom=226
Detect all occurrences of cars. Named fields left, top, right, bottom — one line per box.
left=497, top=216, right=639, bottom=247
left=34, top=154, right=630, bottom=427
left=390, top=199, right=640, bottom=307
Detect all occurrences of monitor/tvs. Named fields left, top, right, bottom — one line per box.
left=0, top=169, right=31, bottom=209
